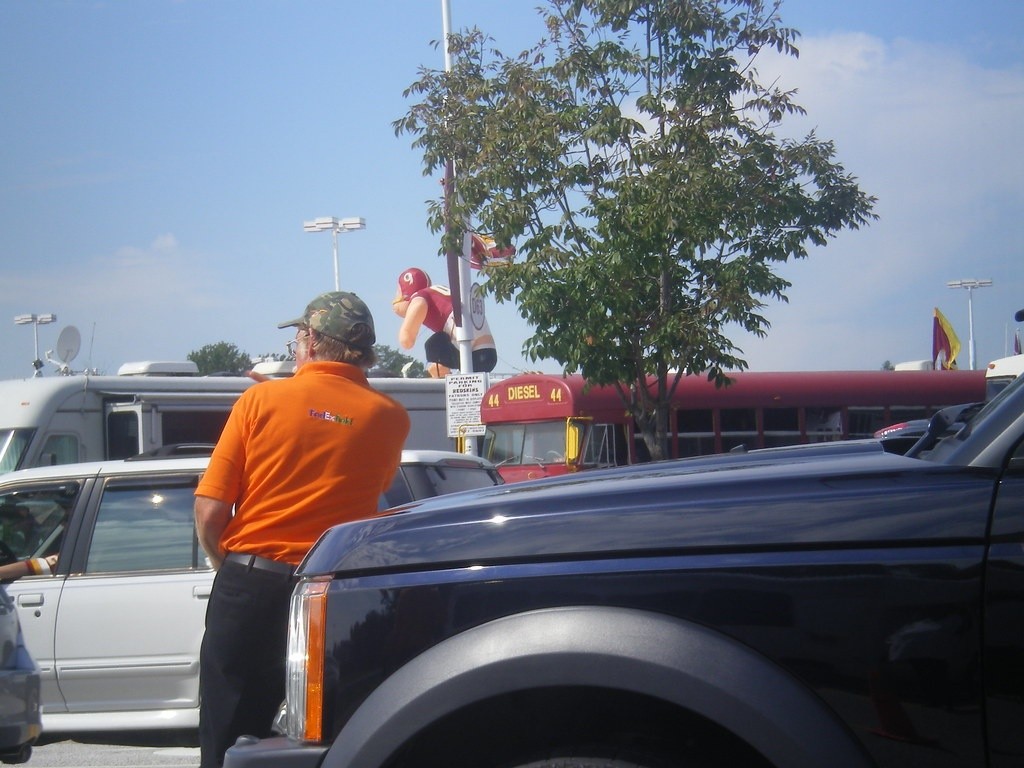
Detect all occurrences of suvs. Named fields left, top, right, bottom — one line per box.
left=0, top=359, right=1024, bottom=768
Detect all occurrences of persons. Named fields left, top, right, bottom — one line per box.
left=0, top=553, right=59, bottom=580
left=193, top=290, right=411, bottom=768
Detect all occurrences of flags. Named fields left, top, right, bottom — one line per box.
left=933, top=308, right=961, bottom=371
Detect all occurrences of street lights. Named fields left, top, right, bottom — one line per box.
left=302, top=215, right=366, bottom=293
left=946, top=277, right=992, bottom=370
left=15, top=313, right=58, bottom=376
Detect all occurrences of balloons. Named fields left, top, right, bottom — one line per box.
left=393, top=269, right=497, bottom=378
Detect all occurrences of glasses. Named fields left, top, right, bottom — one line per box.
left=287, top=333, right=309, bottom=358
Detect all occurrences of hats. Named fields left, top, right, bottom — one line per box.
left=276, top=291, right=375, bottom=347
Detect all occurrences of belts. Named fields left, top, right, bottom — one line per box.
left=225, top=549, right=301, bottom=576
